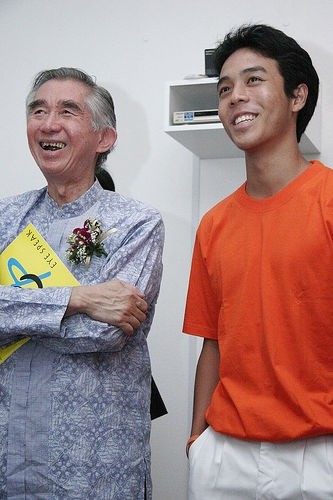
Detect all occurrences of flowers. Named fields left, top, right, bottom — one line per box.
left=65, top=216, right=120, bottom=271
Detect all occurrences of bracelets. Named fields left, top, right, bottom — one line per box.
left=186, top=435, right=200, bottom=457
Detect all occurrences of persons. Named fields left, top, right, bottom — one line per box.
left=0, top=67, right=165, bottom=500
left=183, top=26, right=333, bottom=500
